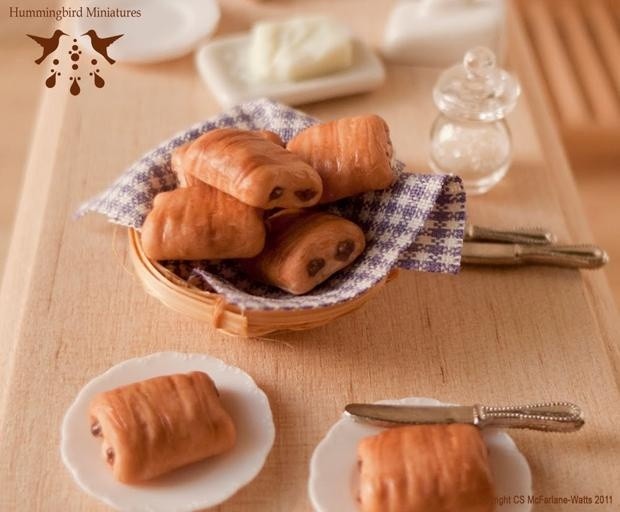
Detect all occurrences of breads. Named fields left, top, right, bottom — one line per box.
left=140, top=113, right=400, bottom=296
left=350, top=420, right=499, bottom=512
left=87, top=371, right=235, bottom=485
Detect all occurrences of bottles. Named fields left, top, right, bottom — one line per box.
left=422, top=44, right=525, bottom=198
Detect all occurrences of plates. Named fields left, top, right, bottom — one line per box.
left=308, top=395, right=535, bottom=512
left=197, top=15, right=385, bottom=107
left=60, top=349, right=276, bottom=512
left=76, top=1, right=222, bottom=64
